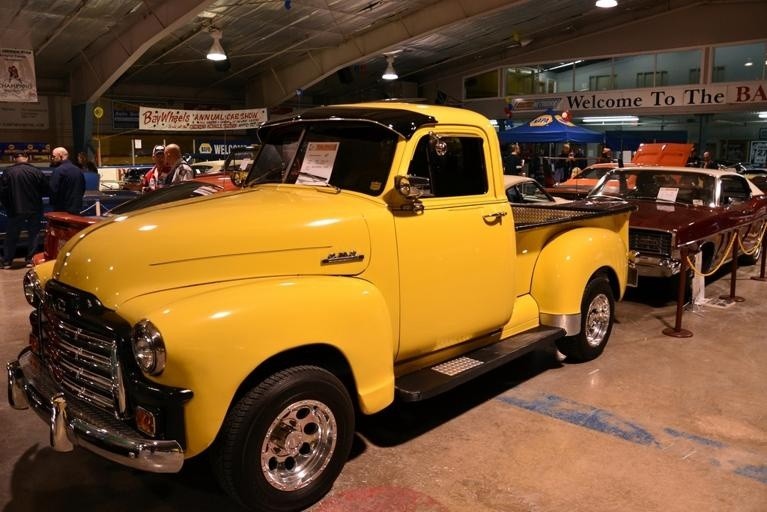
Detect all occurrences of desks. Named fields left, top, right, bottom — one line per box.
left=1, top=190, right=137, bottom=257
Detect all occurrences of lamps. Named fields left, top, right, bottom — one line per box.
left=206, top=30, right=227, bottom=61
left=382, top=56, right=399, bottom=80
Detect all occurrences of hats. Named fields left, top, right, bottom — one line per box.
left=152, top=145, right=165, bottom=156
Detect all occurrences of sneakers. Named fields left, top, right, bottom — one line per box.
left=25, top=258, right=32, bottom=268
left=2, top=259, right=12, bottom=268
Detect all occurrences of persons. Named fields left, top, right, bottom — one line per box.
left=501, top=143, right=612, bottom=185
left=157, top=143, right=195, bottom=189
left=686, top=149, right=700, bottom=167
left=141, top=144, right=170, bottom=193
left=74, top=152, right=98, bottom=174
left=0, top=150, right=48, bottom=269
left=702, top=151, right=715, bottom=169
left=47, top=146, right=86, bottom=216
